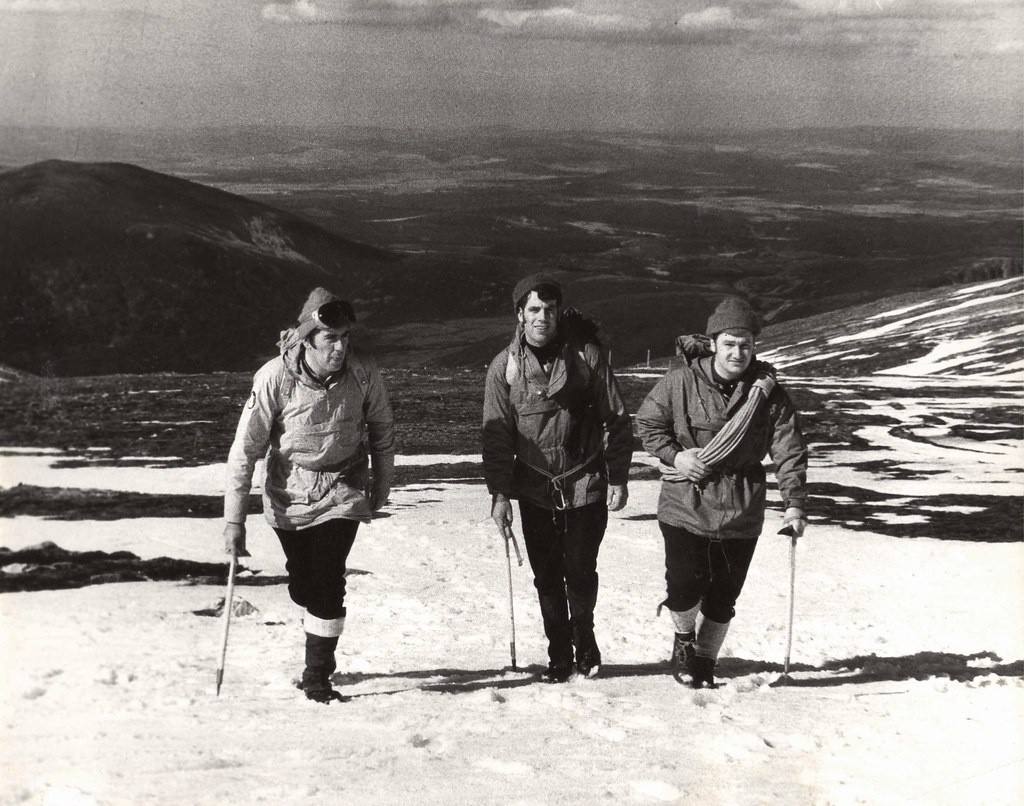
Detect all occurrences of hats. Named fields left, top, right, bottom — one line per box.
left=512, top=273, right=562, bottom=319
left=706, top=298, right=761, bottom=337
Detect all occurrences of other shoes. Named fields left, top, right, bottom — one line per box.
left=695, top=656, right=715, bottom=688
left=672, top=630, right=696, bottom=685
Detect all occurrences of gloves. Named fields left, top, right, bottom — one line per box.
left=225, top=524, right=247, bottom=557
left=366, top=475, right=390, bottom=510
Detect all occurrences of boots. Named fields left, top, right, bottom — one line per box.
left=297, top=607, right=336, bottom=690
left=534, top=577, right=574, bottom=682
left=303, top=608, right=345, bottom=705
left=564, top=572, right=601, bottom=676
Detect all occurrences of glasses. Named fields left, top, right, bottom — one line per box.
left=319, top=300, right=356, bottom=328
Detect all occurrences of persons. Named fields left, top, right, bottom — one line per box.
left=224, top=286, right=396, bottom=704
left=482, top=274, right=634, bottom=684
left=637, top=296, right=810, bottom=688
